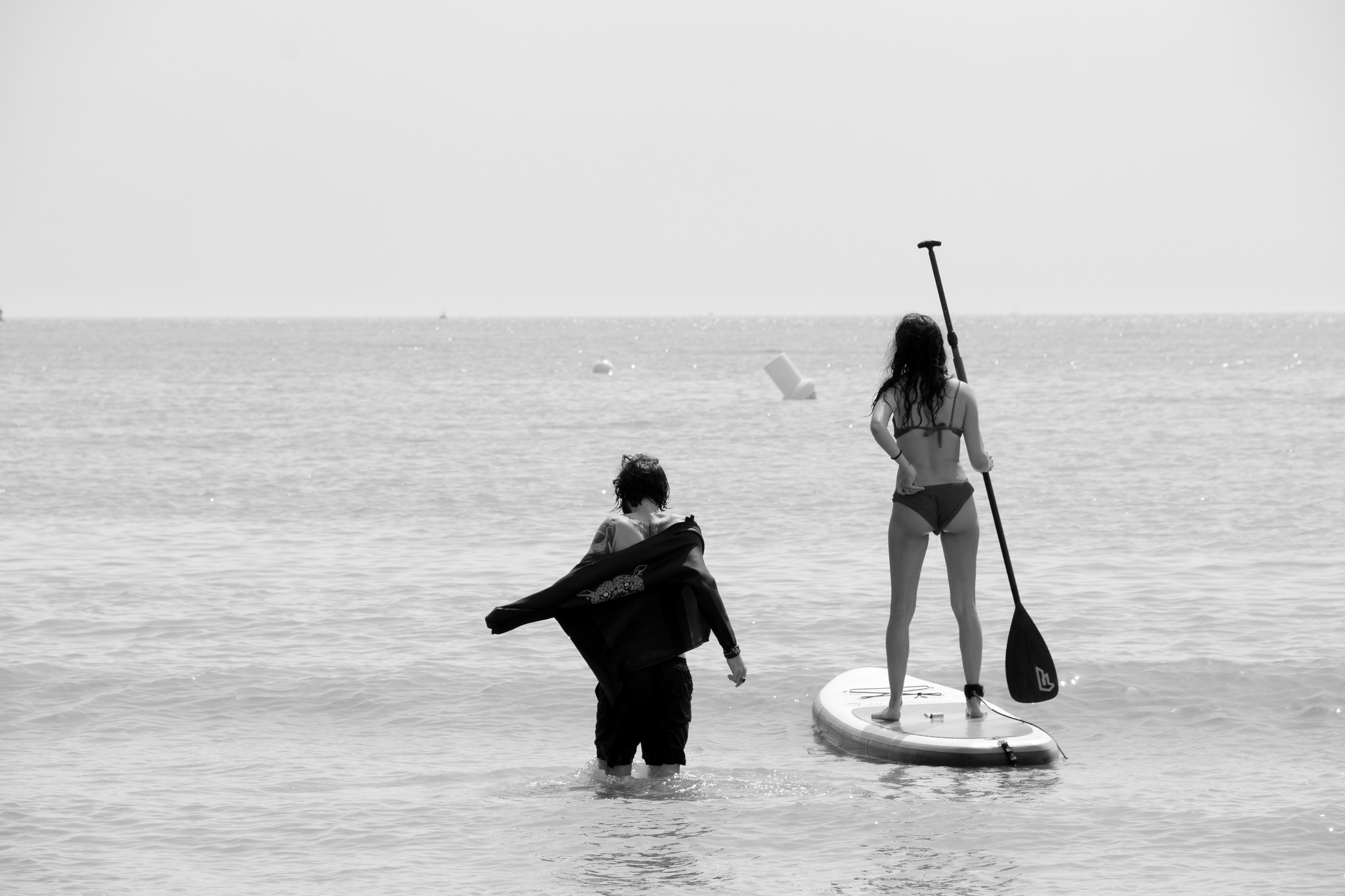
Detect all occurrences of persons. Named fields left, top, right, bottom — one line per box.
left=870, top=313, right=994, bottom=720
left=485, top=454, right=748, bottom=775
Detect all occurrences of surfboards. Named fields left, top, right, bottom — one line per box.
left=808, top=664, right=1059, bottom=772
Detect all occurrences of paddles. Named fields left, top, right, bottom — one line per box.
left=920, top=237, right=1060, bottom=703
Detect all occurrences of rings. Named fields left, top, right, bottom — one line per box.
left=740, top=677, right=746, bottom=683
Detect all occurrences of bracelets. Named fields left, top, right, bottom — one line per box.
left=891, top=449, right=902, bottom=460
